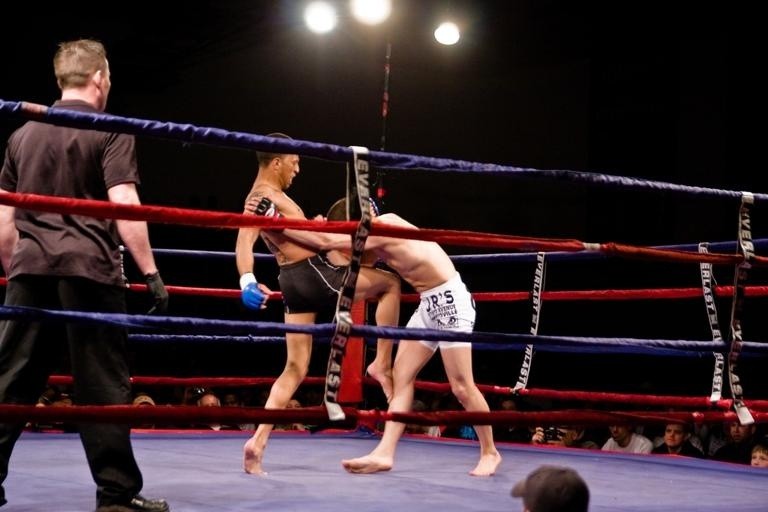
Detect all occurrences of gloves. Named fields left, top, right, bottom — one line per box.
left=254, top=198, right=286, bottom=233
left=146, top=272, right=170, bottom=316
left=239, top=272, right=268, bottom=311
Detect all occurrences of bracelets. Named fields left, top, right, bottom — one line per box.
left=239, top=273, right=257, bottom=291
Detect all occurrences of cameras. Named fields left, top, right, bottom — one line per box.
left=189, top=387, right=206, bottom=403
left=542, top=426, right=564, bottom=441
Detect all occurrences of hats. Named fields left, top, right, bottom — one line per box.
left=510, top=465, right=589, bottom=512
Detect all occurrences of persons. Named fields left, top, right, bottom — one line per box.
left=686, top=422, right=725, bottom=456
left=533, top=425, right=598, bottom=449
left=0, top=38, right=169, bottom=512
left=751, top=444, right=766, bottom=468
left=715, top=422, right=766, bottom=465
left=273, top=395, right=311, bottom=432
left=405, top=400, right=428, bottom=434
left=196, top=395, right=242, bottom=431
left=429, top=398, right=449, bottom=437
left=509, top=464, right=589, bottom=512
left=222, top=394, right=255, bottom=430
left=129, top=393, right=157, bottom=429
left=650, top=423, right=703, bottom=458
left=244, top=194, right=502, bottom=475
left=601, top=423, right=653, bottom=455
left=655, top=425, right=688, bottom=446
left=235, top=132, right=401, bottom=476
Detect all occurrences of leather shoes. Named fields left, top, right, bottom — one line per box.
left=96, top=495, right=169, bottom=512
